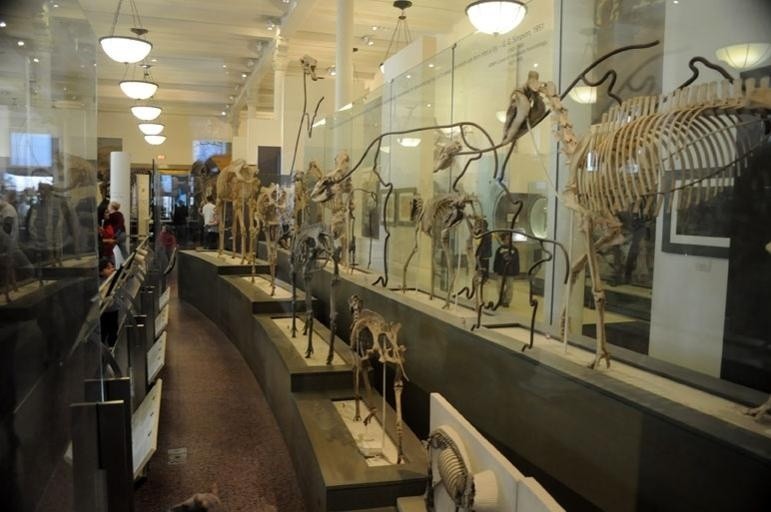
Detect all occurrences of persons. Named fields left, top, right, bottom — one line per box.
left=494, top=234, right=520, bottom=307
left=170, top=196, right=218, bottom=249
left=97, top=170, right=129, bottom=259
left=474, top=221, right=492, bottom=284
left=99, top=259, right=120, bottom=347
